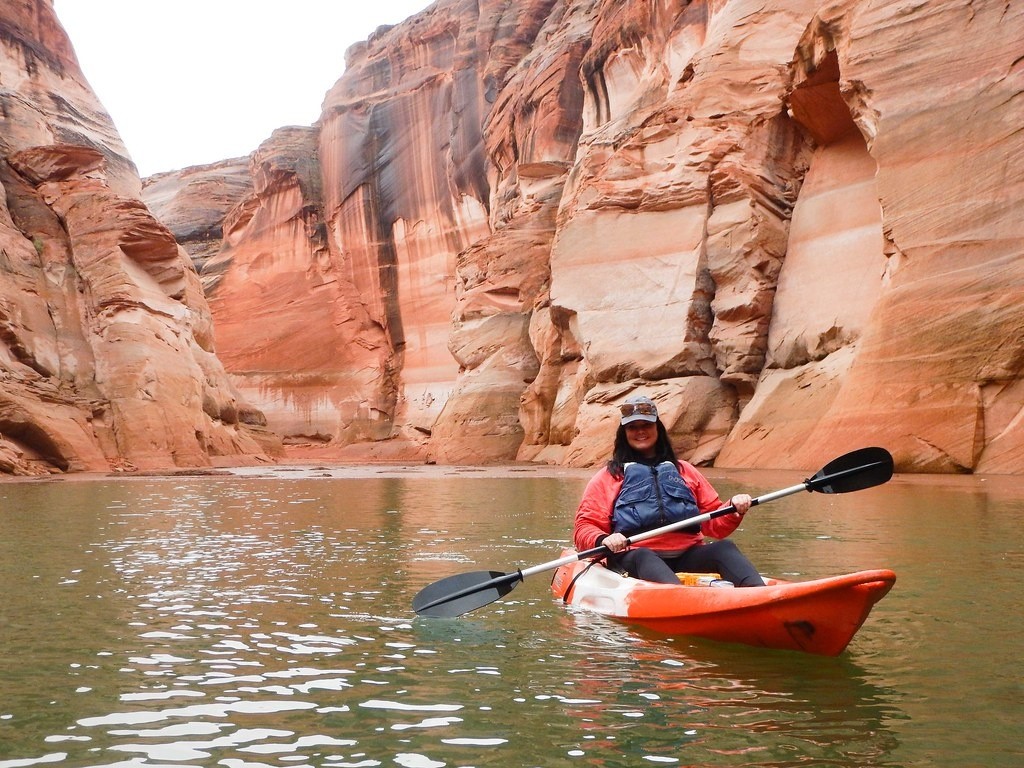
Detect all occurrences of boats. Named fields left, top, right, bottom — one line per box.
left=552, top=543, right=896, bottom=655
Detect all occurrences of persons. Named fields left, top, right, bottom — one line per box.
left=572, top=396, right=766, bottom=588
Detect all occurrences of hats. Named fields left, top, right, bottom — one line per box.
left=621, top=396, right=658, bottom=424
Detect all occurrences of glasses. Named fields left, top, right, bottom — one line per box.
left=620, top=403, right=654, bottom=417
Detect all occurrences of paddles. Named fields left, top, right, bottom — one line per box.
left=410, top=446, right=896, bottom=619
